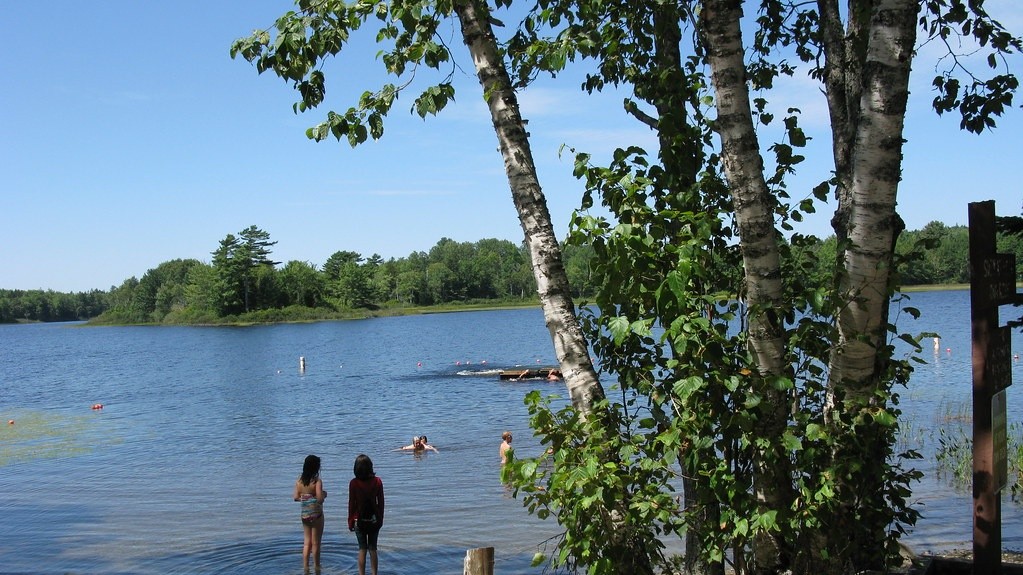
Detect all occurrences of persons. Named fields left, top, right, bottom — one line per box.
left=499, top=431, right=514, bottom=493
left=347, top=454, right=385, bottom=575
left=392, top=435, right=440, bottom=455
left=293, top=454, right=328, bottom=575
